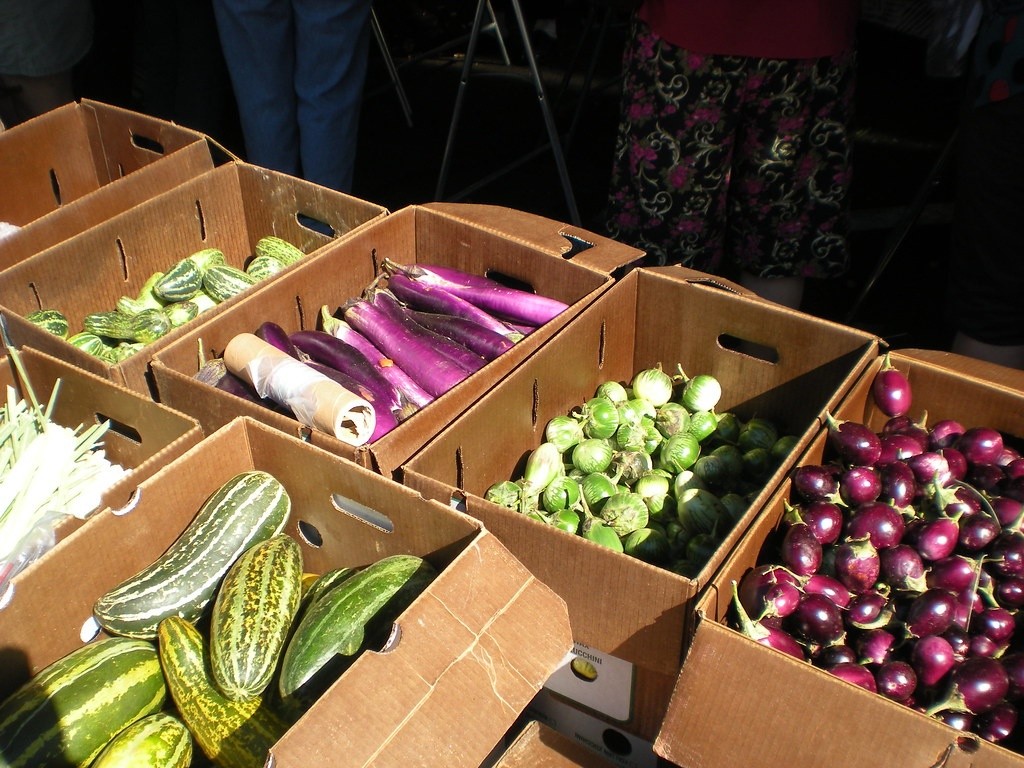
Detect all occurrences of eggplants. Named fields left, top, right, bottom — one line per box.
left=727, top=354, right=1024, bottom=757
left=197, top=253, right=579, bottom=444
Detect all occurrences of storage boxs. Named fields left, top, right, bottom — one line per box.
left=399, top=263, right=891, bottom=746
left=506, top=689, right=661, bottom=768
left=149, top=202, right=648, bottom=486
left=1, top=94, right=246, bottom=275
left=1, top=414, right=573, bottom=768
left=489, top=719, right=624, bottom=768
left=0, top=344, right=209, bottom=606
left=652, top=347, right=1024, bottom=768
left=0, top=161, right=392, bottom=409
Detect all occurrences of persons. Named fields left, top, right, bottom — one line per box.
left=936, top=90, right=1024, bottom=372
left=212, top=0, right=375, bottom=237
left=0, top=0, right=97, bottom=131
left=607, top=0, right=871, bottom=314
